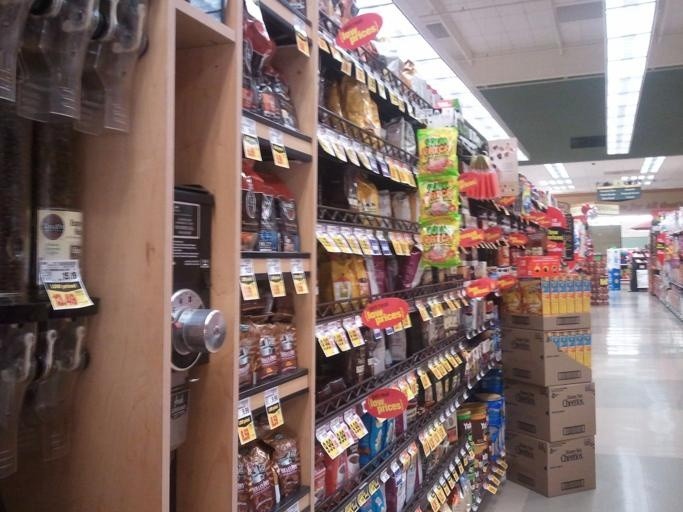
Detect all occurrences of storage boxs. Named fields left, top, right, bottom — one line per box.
left=500, top=328, right=596, bottom=498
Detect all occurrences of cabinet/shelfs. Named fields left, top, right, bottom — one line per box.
left=648, top=229, right=682, bottom=322
left=233, top=0, right=572, bottom=512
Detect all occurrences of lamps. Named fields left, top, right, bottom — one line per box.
left=354, top=0, right=530, bottom=161
left=600, top=0, right=659, bottom=155
